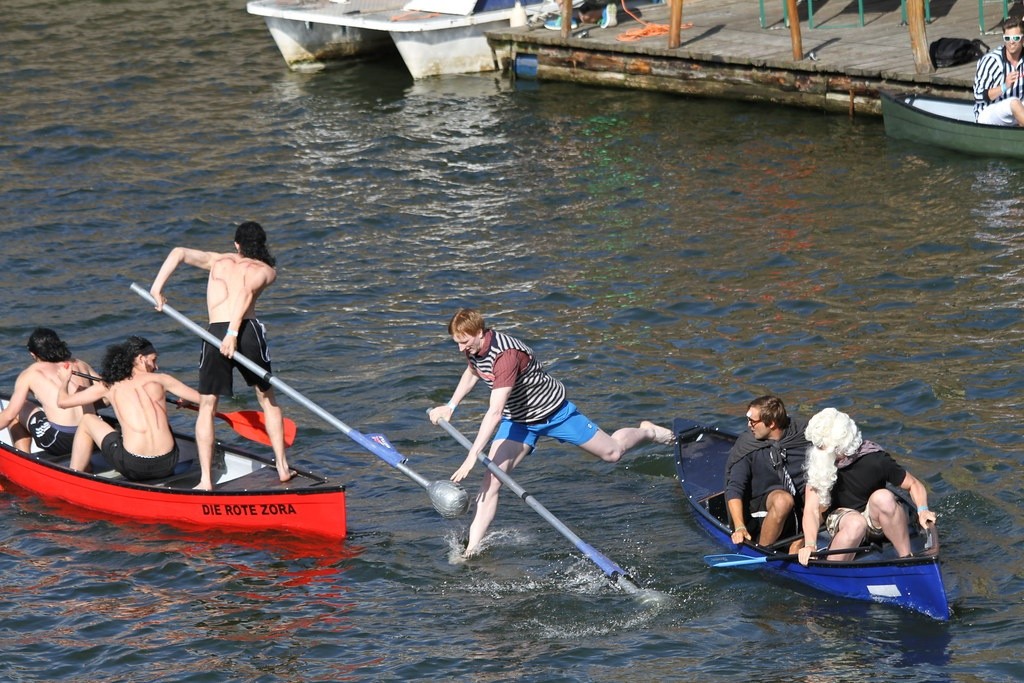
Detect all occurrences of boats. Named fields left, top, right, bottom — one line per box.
left=875, top=84, right=1023, bottom=159
left=671, top=416, right=951, bottom=619
left=0, top=393, right=348, bottom=540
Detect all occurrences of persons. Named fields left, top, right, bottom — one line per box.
left=973, top=17, right=1024, bottom=128
left=0, top=328, right=110, bottom=455
left=543, top=0, right=620, bottom=30
left=726, top=395, right=828, bottom=547
left=797, top=407, right=936, bottom=567
left=56, top=336, right=201, bottom=480
left=429, top=307, right=675, bottom=566
left=150, top=221, right=297, bottom=492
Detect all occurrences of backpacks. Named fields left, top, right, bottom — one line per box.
left=929, top=38, right=990, bottom=69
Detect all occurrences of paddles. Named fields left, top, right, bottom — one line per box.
left=702, top=543, right=885, bottom=567
left=63, top=362, right=297, bottom=449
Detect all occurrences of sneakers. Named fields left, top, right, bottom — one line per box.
left=544, top=17, right=577, bottom=30
left=601, top=3, right=617, bottom=29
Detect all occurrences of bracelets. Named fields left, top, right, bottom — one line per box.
left=917, top=506, right=929, bottom=512
left=447, top=401, right=457, bottom=410
left=226, top=328, right=239, bottom=338
left=1001, top=83, right=1008, bottom=92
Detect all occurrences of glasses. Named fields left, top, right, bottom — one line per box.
left=744, top=415, right=762, bottom=426
left=1003, top=34, right=1024, bottom=42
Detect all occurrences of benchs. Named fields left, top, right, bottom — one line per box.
left=112, top=458, right=222, bottom=485
left=31, top=449, right=101, bottom=461
